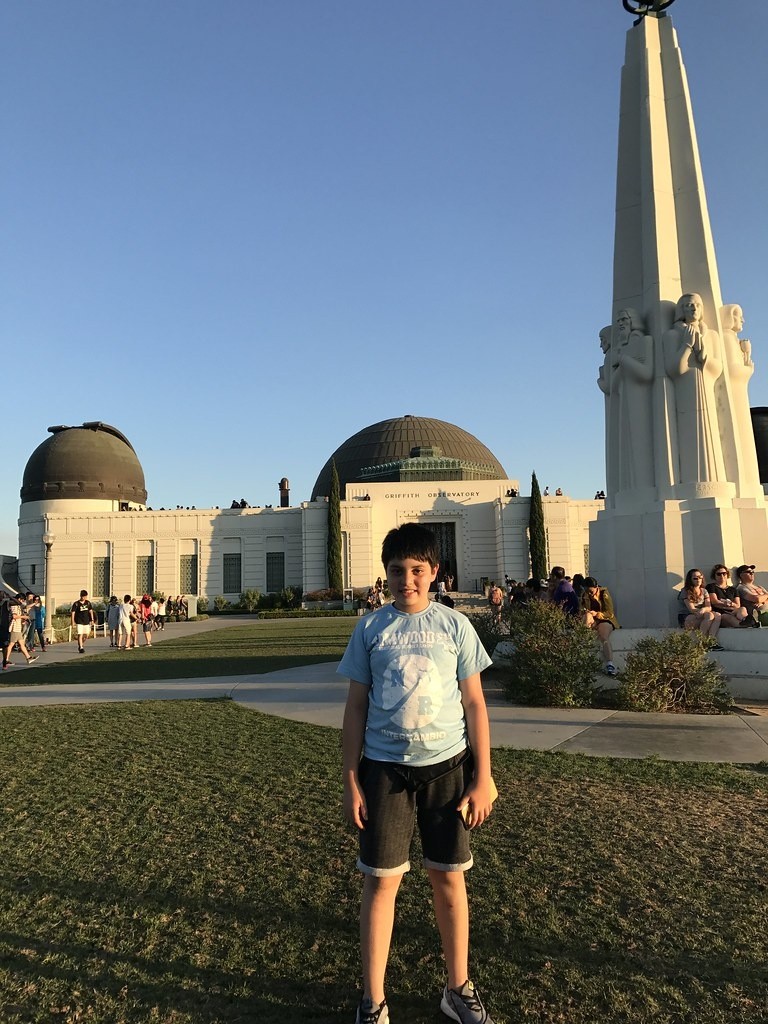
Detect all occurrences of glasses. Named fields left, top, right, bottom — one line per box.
left=715, top=572, right=727, bottom=576
left=548, top=574, right=557, bottom=578
left=742, top=570, right=754, bottom=573
left=691, top=576, right=703, bottom=580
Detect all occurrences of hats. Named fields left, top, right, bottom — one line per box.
left=736, top=565, right=755, bottom=579
left=143, top=595, right=149, bottom=600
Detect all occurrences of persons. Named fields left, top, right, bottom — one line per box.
left=488, top=567, right=619, bottom=675
left=664, top=293, right=727, bottom=482
left=364, top=494, right=370, bottom=501
left=434, top=568, right=455, bottom=609
left=231, top=498, right=249, bottom=508
left=505, top=489, right=518, bottom=497
left=597, top=308, right=653, bottom=510
left=556, top=488, right=563, bottom=496
left=720, top=303, right=764, bottom=497
left=166, top=595, right=187, bottom=616
left=121, top=505, right=219, bottom=511
left=0, top=590, right=47, bottom=670
left=595, top=491, right=605, bottom=499
left=71, top=590, right=94, bottom=654
left=324, top=495, right=328, bottom=501
left=105, top=594, right=166, bottom=650
left=364, top=577, right=386, bottom=614
left=705, top=564, right=768, bottom=628
left=678, top=569, right=725, bottom=651
left=337, top=523, right=493, bottom=1024
left=544, top=487, right=549, bottom=496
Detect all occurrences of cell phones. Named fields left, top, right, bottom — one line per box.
left=459, top=777, right=498, bottom=831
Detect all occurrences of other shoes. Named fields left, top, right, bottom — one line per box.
left=704, top=639, right=725, bottom=652
left=12, top=649, right=17, bottom=651
left=78, top=626, right=166, bottom=653
left=5, top=660, right=15, bottom=666
left=739, top=613, right=756, bottom=627
left=30, top=647, right=36, bottom=652
left=42, top=647, right=47, bottom=652
left=606, top=665, right=615, bottom=675
left=2, top=665, right=8, bottom=670
left=753, top=621, right=761, bottom=628
left=27, top=655, right=39, bottom=664
left=18, top=648, right=22, bottom=653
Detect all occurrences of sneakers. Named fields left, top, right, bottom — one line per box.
left=356, top=998, right=389, bottom=1024
left=440, top=980, right=495, bottom=1024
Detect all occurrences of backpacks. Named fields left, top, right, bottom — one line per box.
left=490, top=587, right=500, bottom=604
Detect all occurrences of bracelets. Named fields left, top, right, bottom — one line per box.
left=91, top=620, right=94, bottom=621
